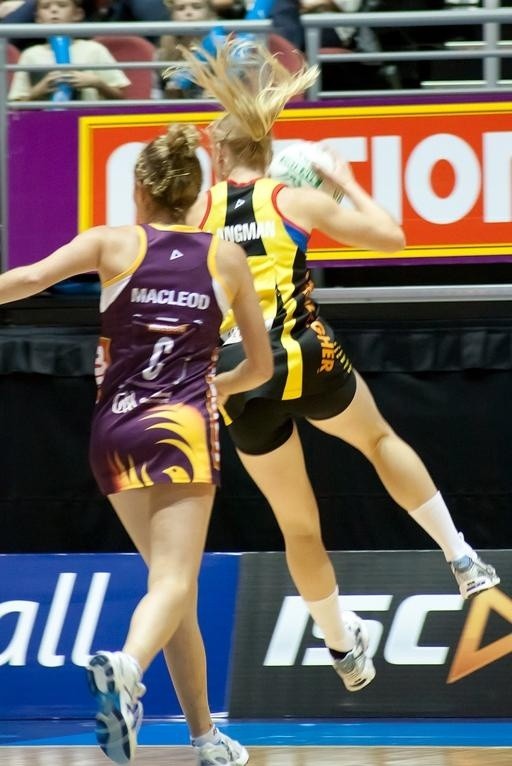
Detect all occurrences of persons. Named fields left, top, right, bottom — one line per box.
left=161, top=29, right=501, bottom=692
left=0, top=124, right=275, bottom=766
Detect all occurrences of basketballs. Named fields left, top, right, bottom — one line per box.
left=268, top=141, right=346, bottom=203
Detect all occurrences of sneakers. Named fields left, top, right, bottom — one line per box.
left=86, top=649, right=146, bottom=765
left=449, top=550, right=500, bottom=601
left=326, top=611, right=378, bottom=694
left=194, top=734, right=252, bottom=766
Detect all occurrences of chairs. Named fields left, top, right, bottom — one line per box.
left=4, top=39, right=359, bottom=111
